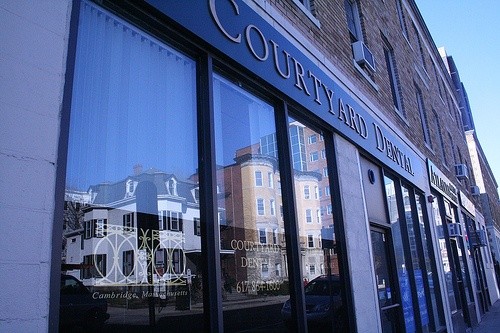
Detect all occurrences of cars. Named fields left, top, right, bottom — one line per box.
left=279, top=274, right=351, bottom=326
left=60, top=274, right=111, bottom=326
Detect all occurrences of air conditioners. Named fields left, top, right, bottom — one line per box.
left=449, top=221, right=463, bottom=239
left=471, top=185, right=480, bottom=198
left=470, top=228, right=488, bottom=248
left=454, top=163, right=469, bottom=181
left=352, top=40, right=375, bottom=74
